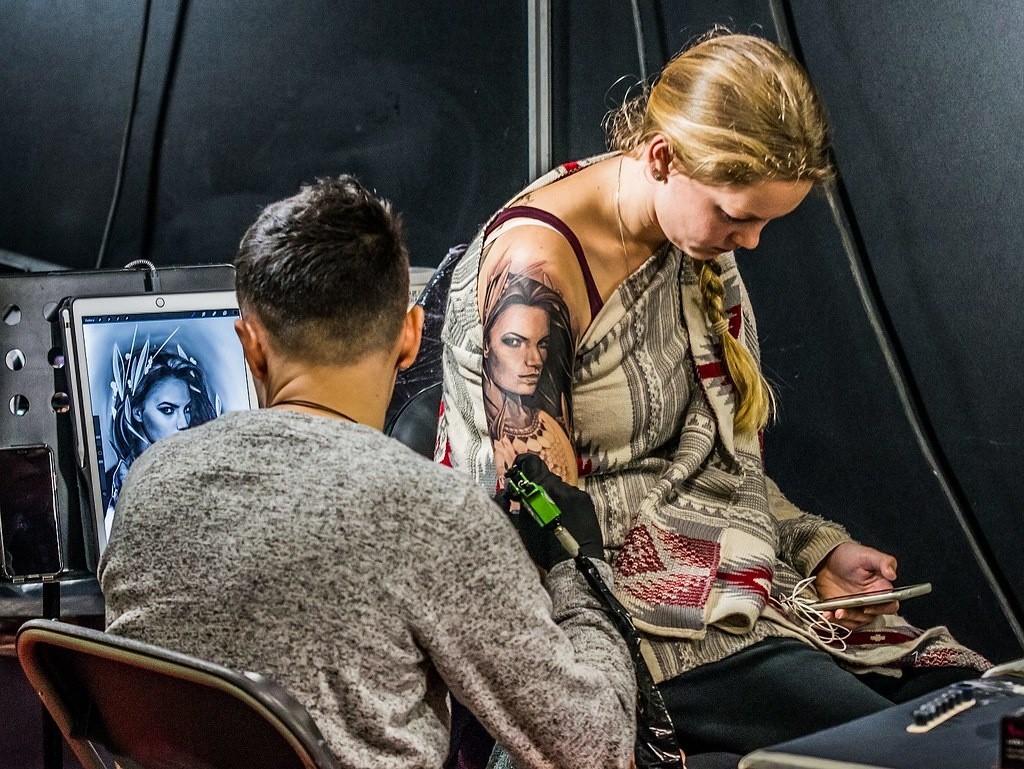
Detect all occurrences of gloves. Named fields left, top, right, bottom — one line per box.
left=492, top=455, right=605, bottom=563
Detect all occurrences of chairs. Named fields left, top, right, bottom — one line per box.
left=12, top=618, right=345, bottom=769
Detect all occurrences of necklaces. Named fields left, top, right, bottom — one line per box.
left=616, top=154, right=631, bottom=278
left=266, top=400, right=361, bottom=425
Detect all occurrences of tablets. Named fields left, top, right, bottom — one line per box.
left=61, top=290, right=259, bottom=573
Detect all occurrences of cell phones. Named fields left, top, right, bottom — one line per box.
left=809, top=582, right=932, bottom=612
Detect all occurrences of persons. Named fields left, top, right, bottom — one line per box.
left=96, top=171, right=640, bottom=769
left=103, top=321, right=220, bottom=545
left=435, top=25, right=997, bottom=769
